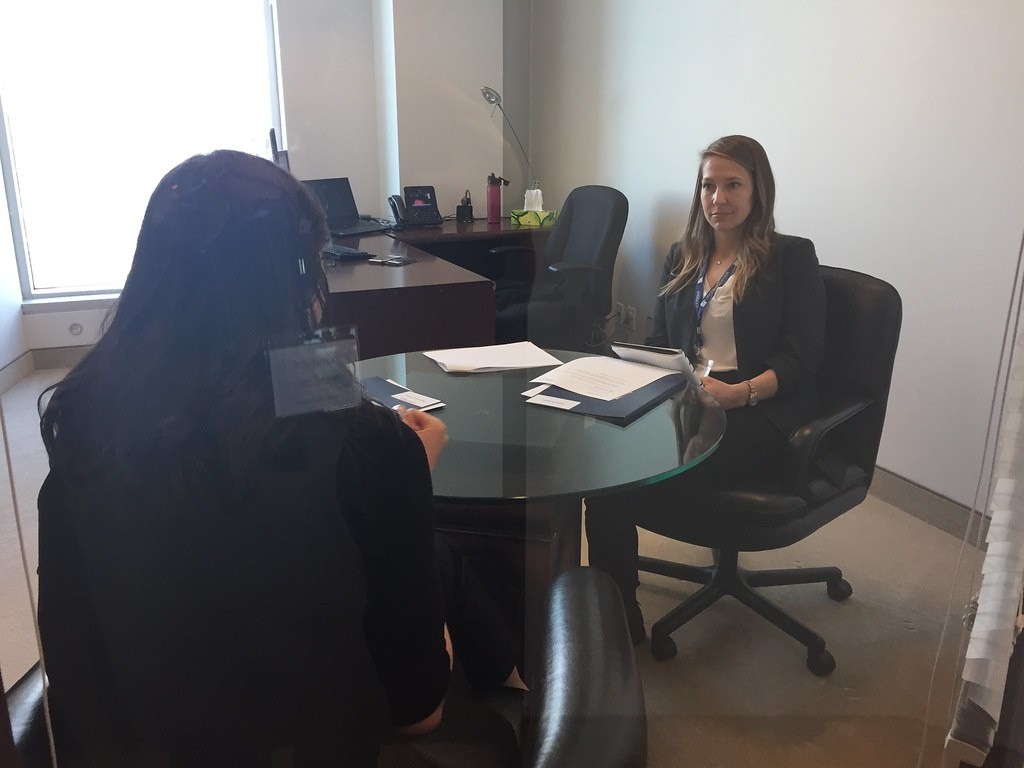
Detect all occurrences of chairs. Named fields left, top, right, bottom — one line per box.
left=633, top=264, right=902, bottom=679
left=484, top=185, right=630, bottom=355
left=0, top=565, right=650, bottom=768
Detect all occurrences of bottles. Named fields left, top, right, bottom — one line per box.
left=486, top=172, right=511, bottom=224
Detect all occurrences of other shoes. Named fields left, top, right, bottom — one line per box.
left=621, top=600, right=647, bottom=644
left=501, top=687, right=539, bottom=708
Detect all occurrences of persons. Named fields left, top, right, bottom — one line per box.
left=37, top=150, right=532, bottom=767
left=559, top=134, right=824, bottom=647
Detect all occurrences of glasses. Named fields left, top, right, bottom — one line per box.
left=318, top=249, right=341, bottom=269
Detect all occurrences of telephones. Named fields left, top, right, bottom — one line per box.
left=387, top=185, right=444, bottom=230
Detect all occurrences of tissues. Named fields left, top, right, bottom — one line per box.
left=511, top=189, right=558, bottom=227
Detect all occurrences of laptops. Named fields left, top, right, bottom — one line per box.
left=301, top=177, right=392, bottom=237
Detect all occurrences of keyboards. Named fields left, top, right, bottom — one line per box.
left=321, top=244, right=377, bottom=261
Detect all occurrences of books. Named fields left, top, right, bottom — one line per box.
left=521, top=357, right=688, bottom=429
left=361, top=375, right=447, bottom=415
left=609, top=341, right=705, bottom=397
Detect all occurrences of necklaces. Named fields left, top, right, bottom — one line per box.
left=710, top=252, right=734, bottom=265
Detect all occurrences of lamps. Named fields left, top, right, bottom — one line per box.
left=479, top=84, right=558, bottom=228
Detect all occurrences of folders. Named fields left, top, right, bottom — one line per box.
left=360, top=375, right=442, bottom=411
left=524, top=374, right=688, bottom=428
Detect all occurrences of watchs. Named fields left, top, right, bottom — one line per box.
left=742, top=379, right=758, bottom=407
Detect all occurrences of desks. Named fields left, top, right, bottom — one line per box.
left=344, top=348, right=728, bottom=590
left=307, top=217, right=555, bottom=364
